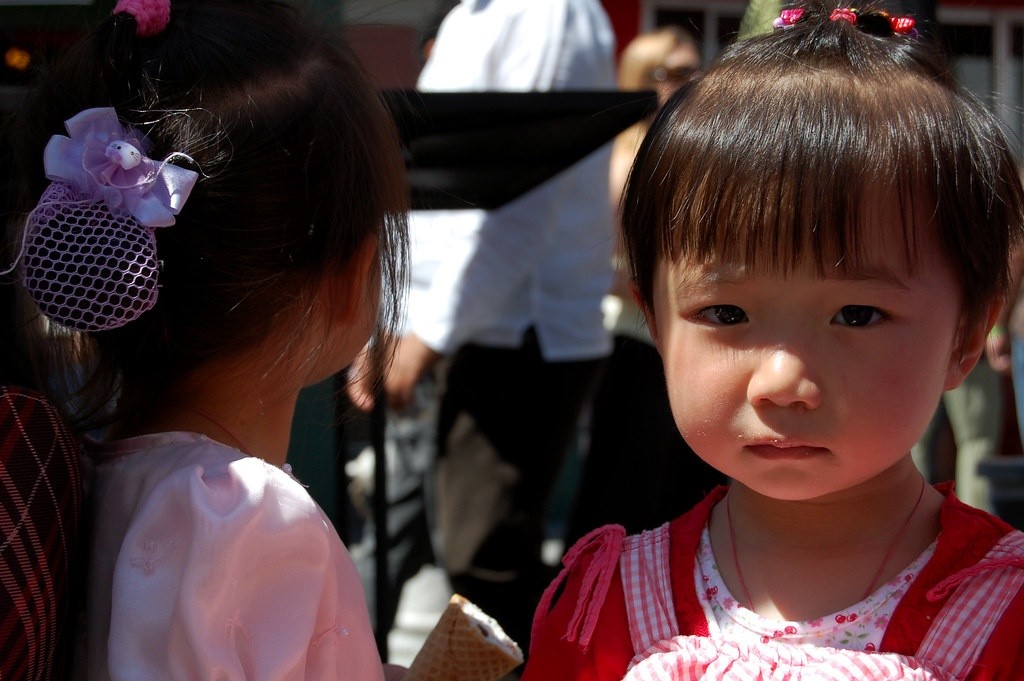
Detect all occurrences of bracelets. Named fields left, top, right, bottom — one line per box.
left=986, top=322, right=1008, bottom=338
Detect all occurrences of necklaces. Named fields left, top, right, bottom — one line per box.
left=726, top=474, right=925, bottom=612
left=187, top=406, right=253, bottom=455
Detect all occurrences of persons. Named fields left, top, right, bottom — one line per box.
left=984, top=167, right=1024, bottom=452
left=346, top=0, right=702, bottom=663
left=13, top=1, right=411, bottom=681
left=518, top=2, right=1024, bottom=681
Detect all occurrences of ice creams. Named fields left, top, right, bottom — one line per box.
left=399, top=594, right=524, bottom=681
